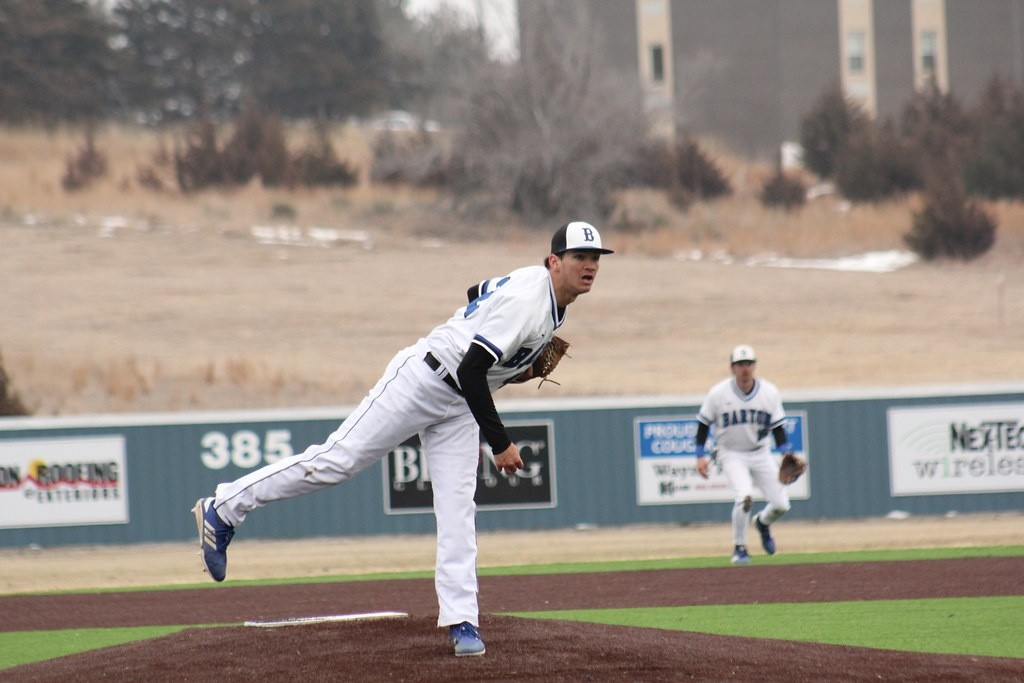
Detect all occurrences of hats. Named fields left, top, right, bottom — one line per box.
left=730, top=345, right=755, bottom=364
left=549, top=221, right=615, bottom=257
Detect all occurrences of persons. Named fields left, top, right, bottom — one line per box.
left=192, top=220, right=615, bottom=656
left=694, top=344, right=796, bottom=564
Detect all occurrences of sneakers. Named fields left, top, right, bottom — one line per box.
left=730, top=551, right=749, bottom=563
left=449, top=620, right=486, bottom=657
left=752, top=511, right=776, bottom=555
left=190, top=495, right=236, bottom=584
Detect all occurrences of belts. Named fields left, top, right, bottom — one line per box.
left=424, top=351, right=465, bottom=397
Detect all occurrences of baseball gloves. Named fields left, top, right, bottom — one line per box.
left=508, top=336, right=573, bottom=390
left=777, top=453, right=807, bottom=485
